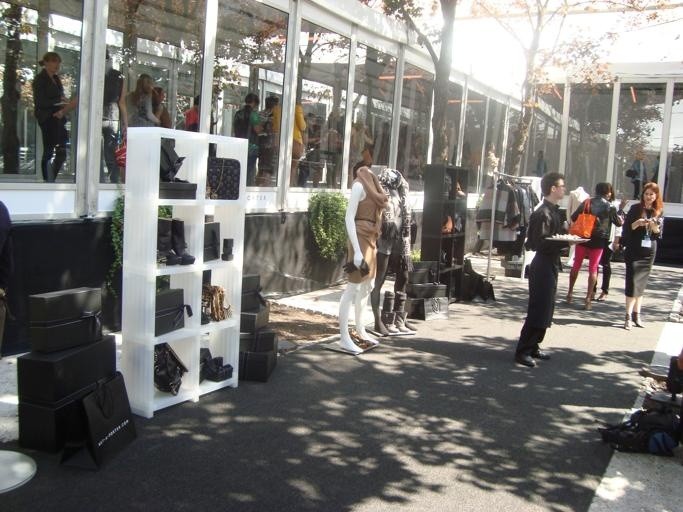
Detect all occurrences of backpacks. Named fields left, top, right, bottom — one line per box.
left=599, top=409, right=681, bottom=455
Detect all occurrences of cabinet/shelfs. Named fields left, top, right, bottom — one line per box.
left=120, top=127, right=248, bottom=419
left=421, top=163, right=469, bottom=305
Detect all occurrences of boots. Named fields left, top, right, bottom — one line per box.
left=158, top=217, right=195, bottom=264
left=382, top=291, right=410, bottom=332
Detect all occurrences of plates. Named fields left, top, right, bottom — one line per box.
left=544, top=237, right=591, bottom=244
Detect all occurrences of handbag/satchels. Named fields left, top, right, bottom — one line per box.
left=202, top=284, right=226, bottom=321
left=154, top=343, right=187, bottom=395
left=425, top=297, right=449, bottom=319
left=572, top=199, right=596, bottom=238
left=84, top=374, right=136, bottom=461
left=161, top=141, right=185, bottom=181
left=207, top=157, right=240, bottom=198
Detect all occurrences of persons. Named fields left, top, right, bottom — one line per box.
left=348, top=122, right=425, bottom=187
left=32, top=48, right=128, bottom=184
left=621, top=182, right=664, bottom=330
left=338, top=167, right=378, bottom=352
left=652, top=156, right=668, bottom=203
left=515, top=172, right=568, bottom=367
left=453, top=143, right=546, bottom=186
left=626, top=151, right=648, bottom=200
left=370, top=168, right=417, bottom=336
left=586, top=183, right=629, bottom=302
left=234, top=94, right=281, bottom=186
left=127, top=74, right=200, bottom=132
left=292, top=105, right=344, bottom=187
left=565, top=183, right=624, bottom=310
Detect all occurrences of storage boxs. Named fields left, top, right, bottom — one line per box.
left=239, top=275, right=277, bottom=383
left=19, top=288, right=117, bottom=455
left=405, top=260, right=448, bottom=322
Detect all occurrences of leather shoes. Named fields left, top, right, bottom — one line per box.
left=625, top=312, right=643, bottom=330
left=517, top=347, right=549, bottom=366
left=568, top=294, right=606, bottom=309
